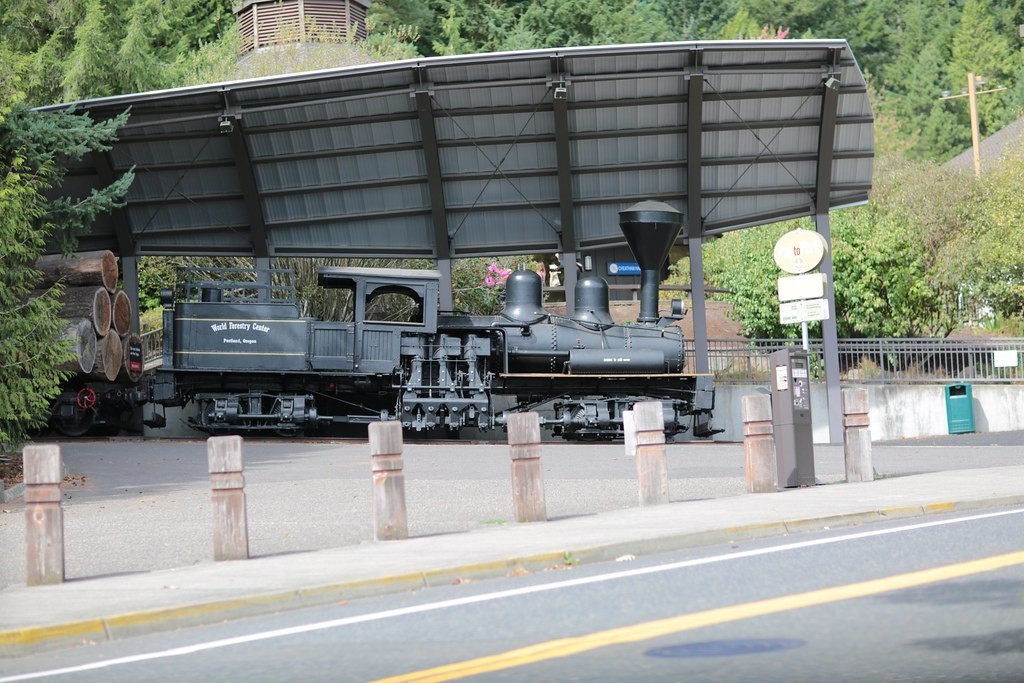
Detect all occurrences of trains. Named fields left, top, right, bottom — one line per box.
left=0, top=199, right=726, bottom=442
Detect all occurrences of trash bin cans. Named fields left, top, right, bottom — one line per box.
left=944, top=383, right=975, bottom=434
